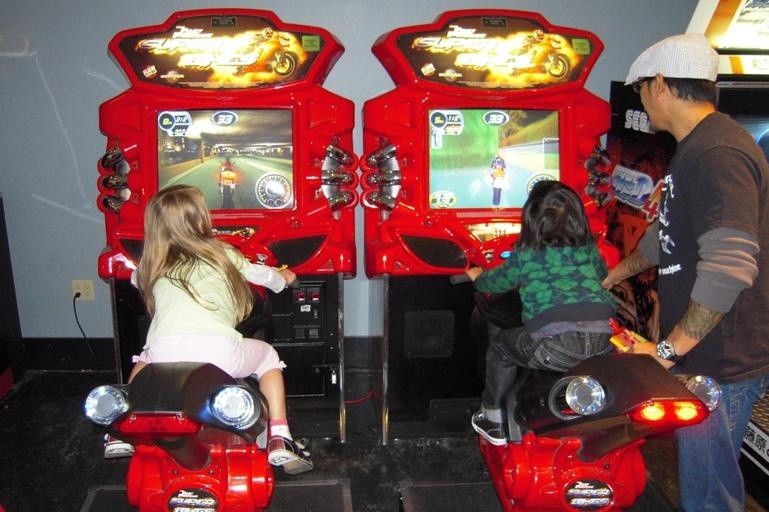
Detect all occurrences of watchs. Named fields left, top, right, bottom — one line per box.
left=656, top=340, right=684, bottom=362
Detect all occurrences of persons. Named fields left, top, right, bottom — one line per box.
left=598, top=34, right=767, bottom=512
left=463, top=178, right=618, bottom=448
left=102, top=184, right=315, bottom=476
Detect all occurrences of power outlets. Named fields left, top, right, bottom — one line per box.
left=72, top=279, right=95, bottom=300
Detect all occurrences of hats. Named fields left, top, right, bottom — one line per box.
left=623, top=31, right=720, bottom=86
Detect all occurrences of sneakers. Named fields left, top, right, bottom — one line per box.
left=102, top=432, right=135, bottom=462
left=266, top=434, right=315, bottom=475
left=470, top=409, right=509, bottom=447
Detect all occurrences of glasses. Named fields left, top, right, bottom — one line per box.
left=631, top=78, right=655, bottom=93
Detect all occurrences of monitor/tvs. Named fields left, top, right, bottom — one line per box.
left=155, top=109, right=295, bottom=211
left=426, top=107, right=561, bottom=210
left=726, top=110, right=768, bottom=165
